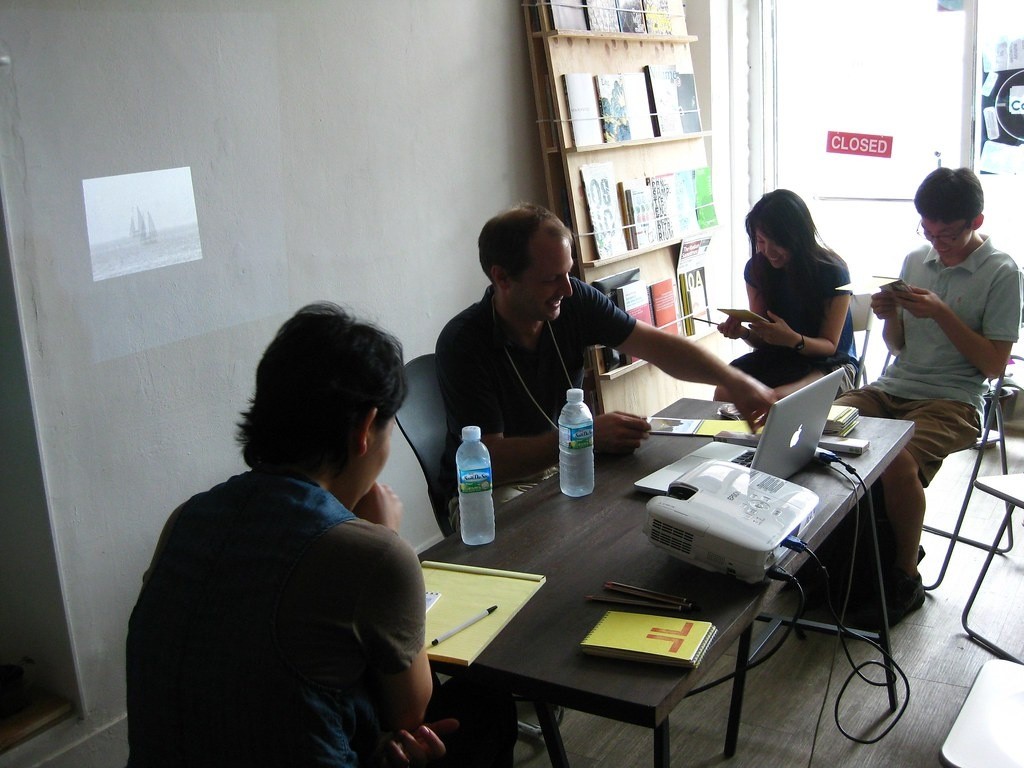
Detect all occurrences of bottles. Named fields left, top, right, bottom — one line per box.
left=559, top=389, right=595, bottom=497
left=456, top=426, right=495, bottom=545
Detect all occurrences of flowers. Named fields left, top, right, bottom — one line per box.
left=989, top=355, right=1024, bottom=395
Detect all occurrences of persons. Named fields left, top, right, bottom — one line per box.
left=435, top=201, right=782, bottom=538
left=831, top=162, right=1021, bottom=633
left=708, top=185, right=864, bottom=409
left=125, top=300, right=520, bottom=768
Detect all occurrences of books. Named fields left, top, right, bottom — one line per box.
left=561, top=63, right=703, bottom=147
left=547, top=0, right=688, bottom=34
left=591, top=231, right=714, bottom=372
left=643, top=416, right=766, bottom=437
left=717, top=308, right=771, bottom=324
left=419, top=560, right=547, bottom=667
left=579, top=160, right=719, bottom=260
left=822, top=405, right=861, bottom=437
left=577, top=610, right=719, bottom=670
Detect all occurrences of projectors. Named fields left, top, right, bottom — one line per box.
left=641, top=458, right=820, bottom=583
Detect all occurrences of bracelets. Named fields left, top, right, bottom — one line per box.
left=741, top=328, right=751, bottom=340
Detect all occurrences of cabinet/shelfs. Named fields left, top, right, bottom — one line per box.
left=519, top=0, right=732, bottom=420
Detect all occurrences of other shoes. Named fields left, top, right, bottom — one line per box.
left=846, top=565, right=926, bottom=633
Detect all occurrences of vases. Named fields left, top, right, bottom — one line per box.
left=983, top=390, right=1018, bottom=422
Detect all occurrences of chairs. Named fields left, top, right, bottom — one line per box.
left=849, top=272, right=1024, bottom=768
left=395, top=352, right=564, bottom=736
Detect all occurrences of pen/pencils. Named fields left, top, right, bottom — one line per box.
left=430, top=605, right=499, bottom=648
left=583, top=580, right=693, bottom=613
left=692, top=316, right=721, bottom=328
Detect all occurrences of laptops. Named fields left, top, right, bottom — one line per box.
left=633, top=367, right=845, bottom=496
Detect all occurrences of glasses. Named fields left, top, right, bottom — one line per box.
left=923, top=221, right=968, bottom=244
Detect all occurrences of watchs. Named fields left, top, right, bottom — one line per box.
left=791, top=334, right=805, bottom=351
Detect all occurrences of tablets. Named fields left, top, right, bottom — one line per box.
left=870, top=276, right=913, bottom=294
left=717, top=308, right=770, bottom=323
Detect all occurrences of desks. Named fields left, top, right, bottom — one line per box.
left=415, top=397, right=916, bottom=768
